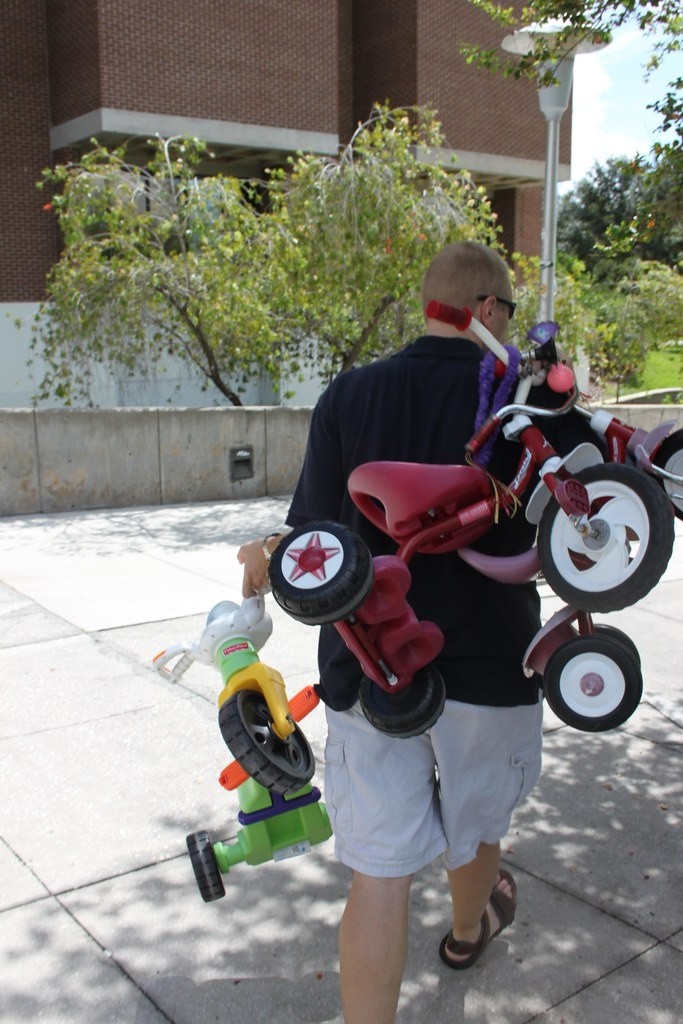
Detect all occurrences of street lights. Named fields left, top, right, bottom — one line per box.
left=501, top=19, right=613, bottom=323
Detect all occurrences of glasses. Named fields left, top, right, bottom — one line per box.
left=477, top=295, right=516, bottom=320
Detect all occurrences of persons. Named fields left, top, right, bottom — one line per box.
left=237, top=241, right=606, bottom=1024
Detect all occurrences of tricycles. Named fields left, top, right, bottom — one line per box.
left=456, top=367, right=683, bottom=734
left=267, top=366, right=683, bottom=739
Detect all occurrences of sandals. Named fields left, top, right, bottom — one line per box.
left=438, top=868, right=517, bottom=971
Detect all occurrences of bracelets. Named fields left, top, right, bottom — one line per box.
left=262, top=532, right=281, bottom=563
